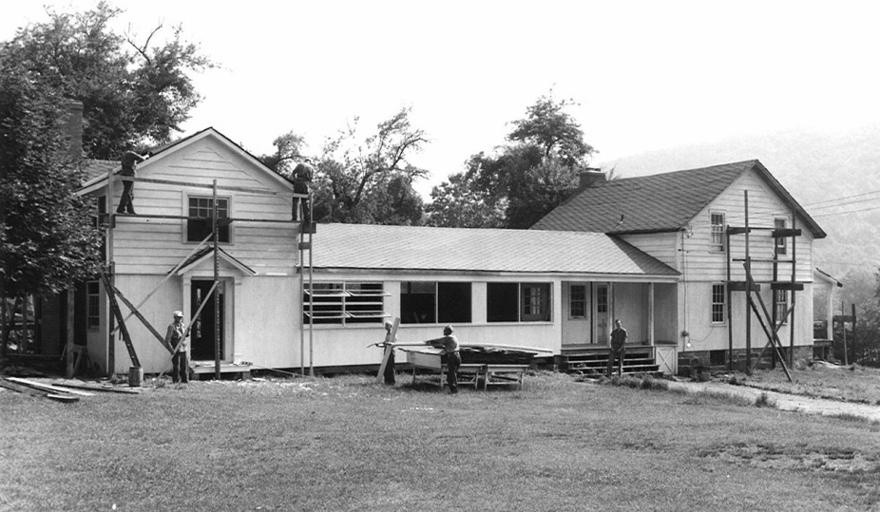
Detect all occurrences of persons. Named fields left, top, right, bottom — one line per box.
left=603, top=318, right=628, bottom=378
left=164, top=311, right=190, bottom=382
left=113, top=138, right=151, bottom=215
left=425, top=325, right=462, bottom=395
left=287, top=157, right=315, bottom=224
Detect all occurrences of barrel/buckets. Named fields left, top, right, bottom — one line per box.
left=129, top=366, right=143, bottom=387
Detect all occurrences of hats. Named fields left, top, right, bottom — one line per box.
left=173, top=310, right=184, bottom=319
